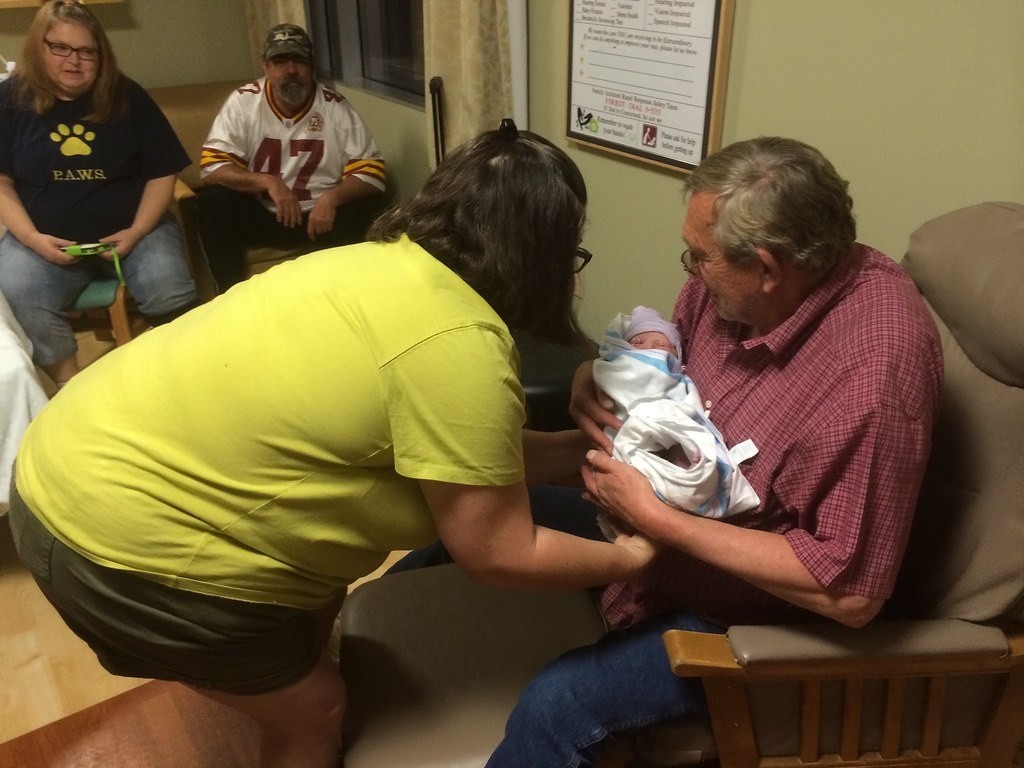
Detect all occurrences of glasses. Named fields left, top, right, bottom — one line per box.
left=680, top=246, right=735, bottom=279
left=43, top=37, right=102, bottom=62
left=570, top=246, right=593, bottom=274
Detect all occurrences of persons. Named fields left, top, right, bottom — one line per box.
left=483, top=135, right=946, bottom=768
left=0, top=0, right=200, bottom=393
left=7, top=120, right=657, bottom=765
left=195, top=23, right=386, bottom=299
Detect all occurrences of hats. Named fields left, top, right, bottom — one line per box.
left=264, top=22, right=312, bottom=60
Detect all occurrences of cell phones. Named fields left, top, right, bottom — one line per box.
left=60, top=243, right=111, bottom=256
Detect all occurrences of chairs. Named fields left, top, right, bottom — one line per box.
left=68, top=278, right=135, bottom=348
left=329, top=195, right=1023, bottom=768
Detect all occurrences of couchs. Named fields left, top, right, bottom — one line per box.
left=140, top=75, right=385, bottom=309
